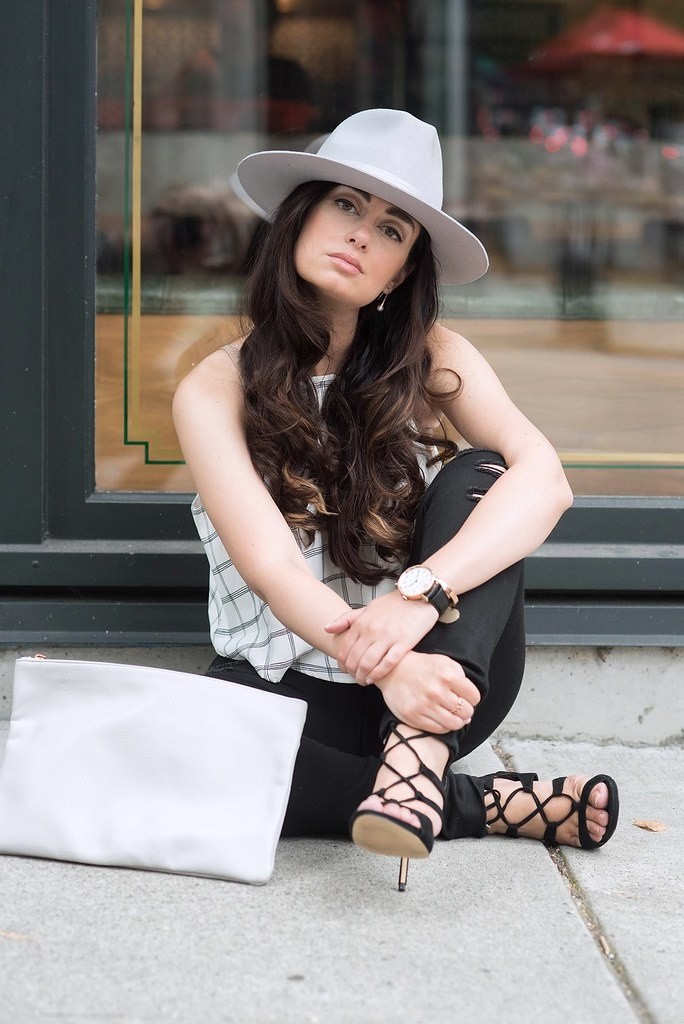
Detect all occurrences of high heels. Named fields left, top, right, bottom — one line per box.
left=348, top=719, right=453, bottom=891
left=476, top=770, right=619, bottom=851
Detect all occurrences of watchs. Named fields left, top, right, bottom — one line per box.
left=395, top=564, right=460, bottom=623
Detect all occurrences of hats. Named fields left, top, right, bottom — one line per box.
left=236, top=109, right=490, bottom=287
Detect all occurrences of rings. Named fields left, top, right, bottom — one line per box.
left=452, top=699, right=464, bottom=714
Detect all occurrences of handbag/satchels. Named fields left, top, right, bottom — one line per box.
left=0, top=658, right=307, bottom=887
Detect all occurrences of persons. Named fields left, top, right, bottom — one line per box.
left=169, top=107, right=618, bottom=894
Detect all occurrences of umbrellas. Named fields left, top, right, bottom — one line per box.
left=529, top=0, right=684, bottom=71
